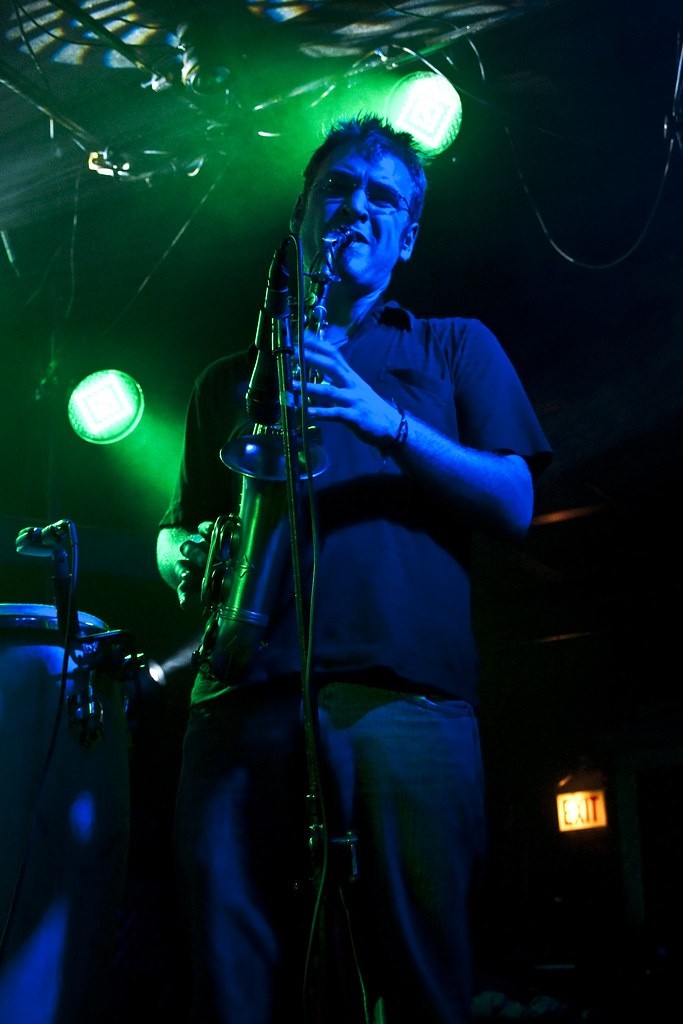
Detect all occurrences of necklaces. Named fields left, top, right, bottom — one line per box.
left=333, top=337, right=348, bottom=345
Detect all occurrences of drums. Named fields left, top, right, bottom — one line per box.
left=1, top=601, right=132, bottom=1023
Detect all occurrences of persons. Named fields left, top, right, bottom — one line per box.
left=156, top=114, right=553, bottom=1024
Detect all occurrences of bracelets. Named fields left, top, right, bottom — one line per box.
left=379, top=398, right=408, bottom=457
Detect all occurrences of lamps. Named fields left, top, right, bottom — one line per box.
left=34, top=350, right=148, bottom=446
left=322, top=69, right=464, bottom=161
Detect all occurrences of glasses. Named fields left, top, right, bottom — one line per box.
left=309, top=176, right=412, bottom=215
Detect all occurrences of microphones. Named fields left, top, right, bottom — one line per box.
left=16, top=518, right=73, bottom=557
left=246, top=247, right=289, bottom=426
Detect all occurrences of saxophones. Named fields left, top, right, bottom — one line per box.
left=188, top=224, right=360, bottom=727
